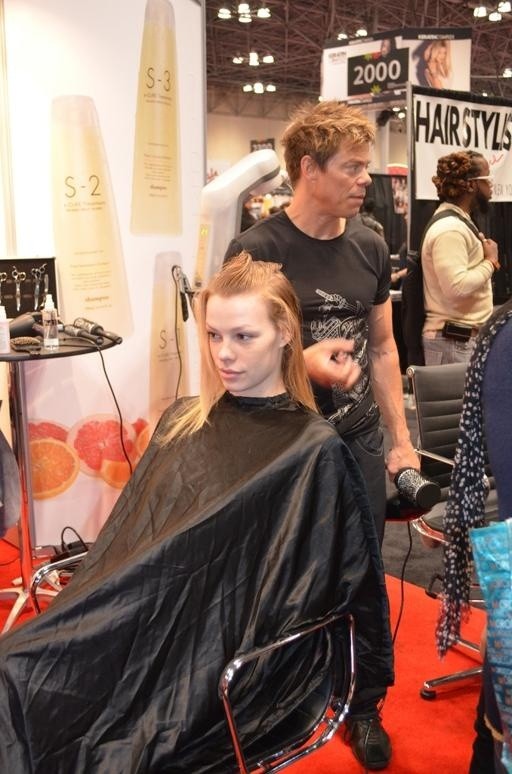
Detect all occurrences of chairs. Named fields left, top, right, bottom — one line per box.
left=408, top=364, right=499, bottom=698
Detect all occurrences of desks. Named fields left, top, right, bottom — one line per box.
left=0, top=334, right=120, bottom=633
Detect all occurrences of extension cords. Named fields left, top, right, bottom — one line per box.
left=51, top=546, right=86, bottom=563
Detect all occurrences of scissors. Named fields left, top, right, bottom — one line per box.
left=0, top=271, right=8, bottom=285
left=29, top=263, right=49, bottom=312
left=10, top=264, right=27, bottom=312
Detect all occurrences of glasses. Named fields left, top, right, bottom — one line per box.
left=471, top=174, right=494, bottom=188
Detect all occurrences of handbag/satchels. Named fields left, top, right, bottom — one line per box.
left=401, top=250, right=425, bottom=365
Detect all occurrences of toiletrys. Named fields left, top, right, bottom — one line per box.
left=40, top=292, right=60, bottom=348
left=0, top=305, right=12, bottom=354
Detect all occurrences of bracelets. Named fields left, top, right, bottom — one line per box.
left=485, top=255, right=500, bottom=269
left=395, top=272, right=402, bottom=281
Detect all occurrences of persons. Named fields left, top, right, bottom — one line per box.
left=420, top=151, right=500, bottom=365
left=1, top=247, right=396, bottom=773
left=389, top=242, right=408, bottom=283
left=381, top=37, right=396, bottom=59
left=424, top=40, right=452, bottom=90
left=223, top=99, right=422, bottom=769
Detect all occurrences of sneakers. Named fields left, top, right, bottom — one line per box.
left=344, top=714, right=391, bottom=770
